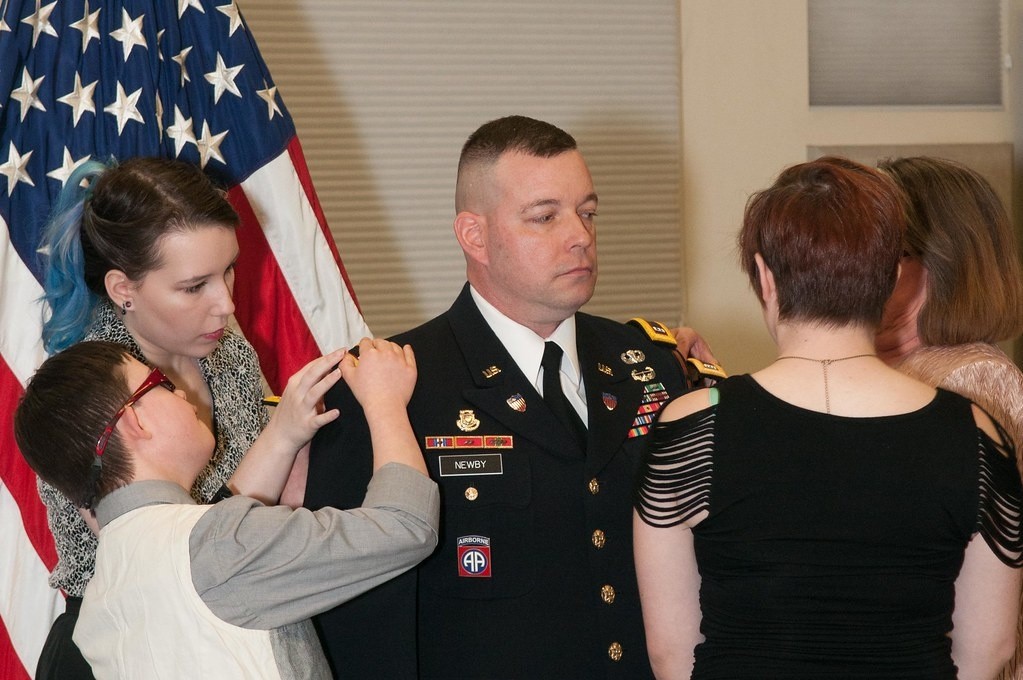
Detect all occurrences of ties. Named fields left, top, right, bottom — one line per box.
left=540, top=340, right=588, bottom=459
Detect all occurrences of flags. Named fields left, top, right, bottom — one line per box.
left=0, top=0, right=376, bottom=679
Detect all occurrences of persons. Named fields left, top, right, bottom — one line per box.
left=14, top=337, right=440, bottom=680
left=35, top=157, right=348, bottom=679
left=667, top=155, right=1023, bottom=482
left=634, top=155, right=1023, bottom=680
left=302, top=115, right=691, bottom=680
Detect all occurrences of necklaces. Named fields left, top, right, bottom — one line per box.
left=775, top=353, right=877, bottom=414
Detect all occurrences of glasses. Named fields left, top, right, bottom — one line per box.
left=90, top=366, right=176, bottom=470
